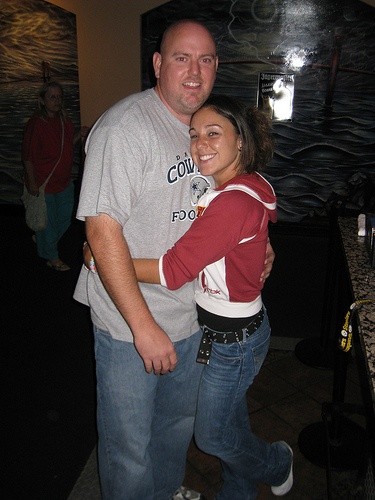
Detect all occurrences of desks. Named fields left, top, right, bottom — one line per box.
left=337, top=216, right=375, bottom=401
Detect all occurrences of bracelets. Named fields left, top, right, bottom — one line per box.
left=90, top=256, right=95, bottom=273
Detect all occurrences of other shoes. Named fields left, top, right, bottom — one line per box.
left=272, top=441, right=293, bottom=495
left=170, top=486, right=201, bottom=500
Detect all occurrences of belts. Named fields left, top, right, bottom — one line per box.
left=196, top=308, right=265, bottom=364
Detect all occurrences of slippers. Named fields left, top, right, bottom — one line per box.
left=47, top=260, right=71, bottom=271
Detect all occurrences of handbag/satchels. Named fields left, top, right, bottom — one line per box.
left=21, top=184, right=48, bottom=232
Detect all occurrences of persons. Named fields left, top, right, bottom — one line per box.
left=83, top=94, right=293, bottom=496
left=73, top=19, right=220, bottom=500
left=22, top=83, right=87, bottom=271
